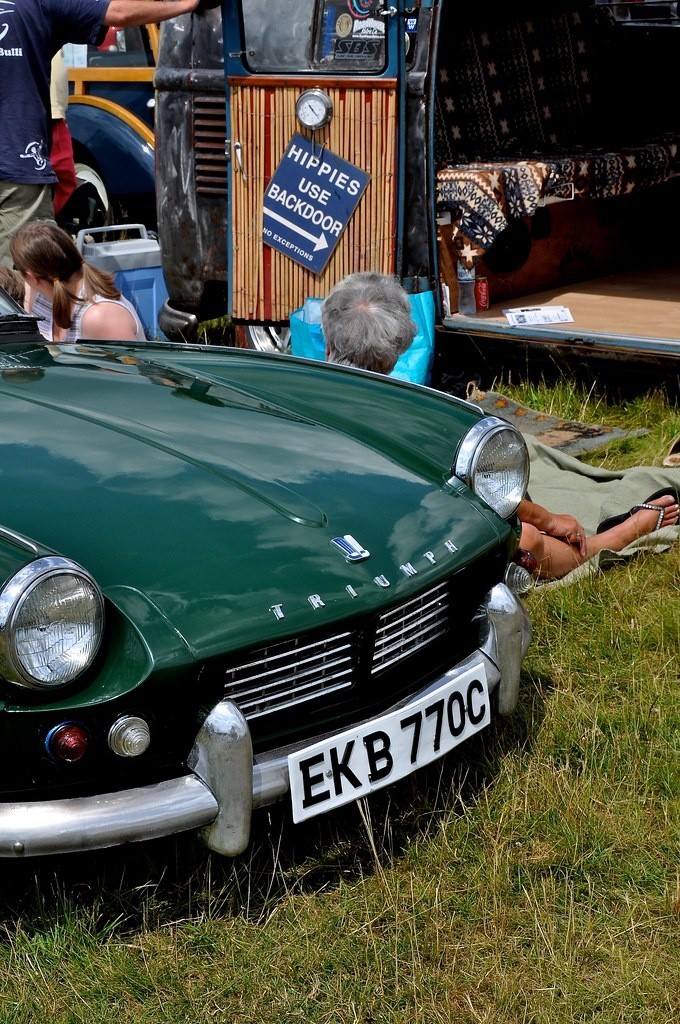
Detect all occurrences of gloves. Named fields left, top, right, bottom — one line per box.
left=191, top=0, right=223, bottom=19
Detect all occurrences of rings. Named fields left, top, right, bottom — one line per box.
left=576, top=532, right=583, bottom=537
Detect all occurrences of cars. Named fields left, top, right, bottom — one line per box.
left=0, top=289, right=535, bottom=861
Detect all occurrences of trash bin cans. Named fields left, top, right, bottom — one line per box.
left=75, top=223, right=172, bottom=342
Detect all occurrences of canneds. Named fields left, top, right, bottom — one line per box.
left=475, top=277, right=489, bottom=310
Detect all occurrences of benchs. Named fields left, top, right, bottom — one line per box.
left=433, top=0, right=680, bottom=317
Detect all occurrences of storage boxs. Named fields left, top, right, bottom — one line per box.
left=71, top=223, right=172, bottom=342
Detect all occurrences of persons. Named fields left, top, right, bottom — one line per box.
left=0, top=0, right=200, bottom=280
left=318, top=271, right=680, bottom=579
left=10, top=224, right=147, bottom=345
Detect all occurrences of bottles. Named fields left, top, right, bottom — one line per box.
left=457, top=263, right=476, bottom=314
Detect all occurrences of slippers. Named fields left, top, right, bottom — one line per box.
left=597, top=487, right=680, bottom=534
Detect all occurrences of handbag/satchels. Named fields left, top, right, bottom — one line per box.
left=289, top=290, right=436, bottom=386
